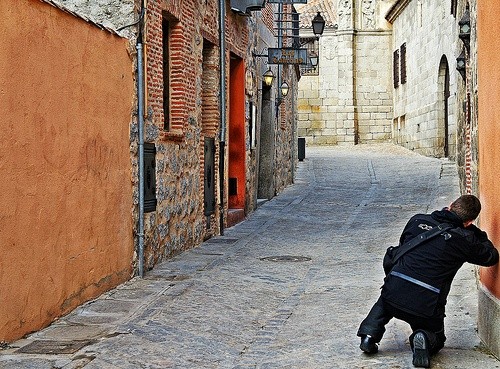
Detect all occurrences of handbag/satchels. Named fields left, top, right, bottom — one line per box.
left=383, top=246, right=399, bottom=277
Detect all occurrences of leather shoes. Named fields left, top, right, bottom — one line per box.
left=413, top=333, right=429, bottom=368
left=360, top=336, right=378, bottom=353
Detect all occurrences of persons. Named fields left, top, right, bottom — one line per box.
left=357, top=195, right=499, bottom=367
